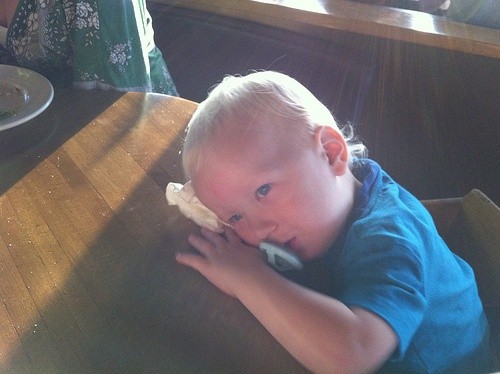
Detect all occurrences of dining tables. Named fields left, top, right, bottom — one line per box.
left=0, top=82, right=340, bottom=374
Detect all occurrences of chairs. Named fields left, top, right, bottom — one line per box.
left=416, top=187, right=500, bottom=338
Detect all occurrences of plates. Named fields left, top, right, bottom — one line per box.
left=0, top=65, right=55, bottom=130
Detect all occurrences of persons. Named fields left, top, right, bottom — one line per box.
left=175, top=71, right=500, bottom=374
left=0, top=0, right=180, bottom=97
